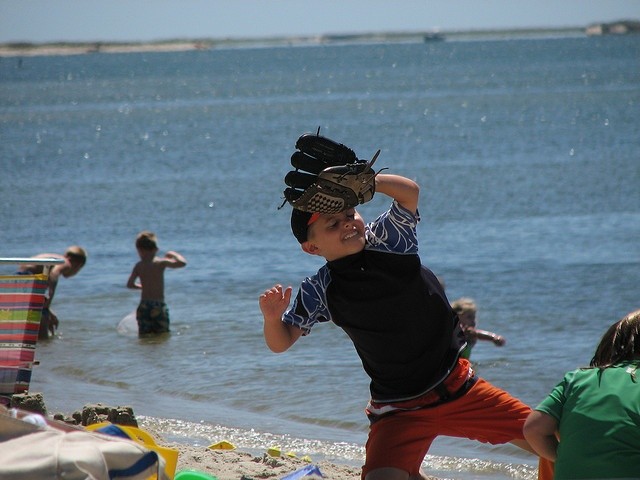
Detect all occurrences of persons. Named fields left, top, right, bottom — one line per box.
left=11, top=246, right=86, bottom=342
left=127, top=231, right=187, bottom=335
left=522, top=309, right=639, bottom=479
left=257, top=131, right=561, bottom=480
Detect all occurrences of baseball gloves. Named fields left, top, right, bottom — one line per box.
left=277, top=126, right=388, bottom=217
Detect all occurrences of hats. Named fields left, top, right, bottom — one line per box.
left=291, top=208, right=321, bottom=235
